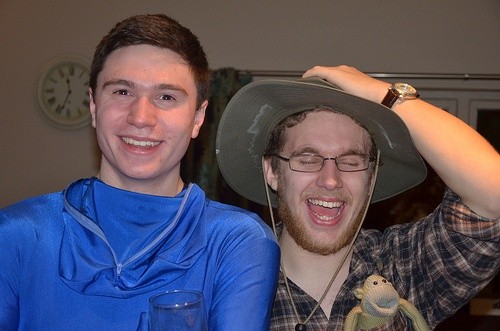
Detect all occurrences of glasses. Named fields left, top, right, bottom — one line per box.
left=269, top=148, right=375, bottom=172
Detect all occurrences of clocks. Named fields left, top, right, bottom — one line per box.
left=34, top=55, right=99, bottom=130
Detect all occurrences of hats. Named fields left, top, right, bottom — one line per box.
left=215, top=76, right=427, bottom=208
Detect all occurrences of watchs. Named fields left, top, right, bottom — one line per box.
left=380, top=81, right=420, bottom=109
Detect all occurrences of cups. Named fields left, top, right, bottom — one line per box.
left=149, top=290, right=208, bottom=331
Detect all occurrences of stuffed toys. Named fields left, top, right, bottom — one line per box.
left=344, top=274, right=431, bottom=331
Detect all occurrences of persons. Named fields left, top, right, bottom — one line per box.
left=0, top=14, right=280, bottom=331
left=216, top=64, right=500, bottom=331
